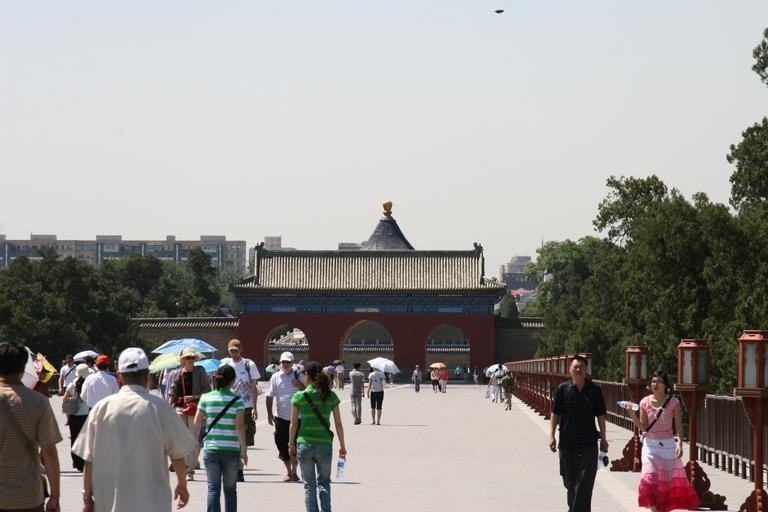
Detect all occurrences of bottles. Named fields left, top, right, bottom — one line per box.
left=599, top=450, right=609, bottom=473
left=617, top=400, right=640, bottom=411
left=335, top=457, right=346, bottom=482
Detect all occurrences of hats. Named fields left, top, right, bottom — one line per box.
left=38, top=365, right=50, bottom=375
left=76, top=363, right=94, bottom=378
left=279, top=352, right=294, bottom=363
left=180, top=348, right=199, bottom=361
left=227, top=340, right=241, bottom=351
left=301, top=361, right=323, bottom=374
left=118, top=348, right=148, bottom=372
left=96, top=355, right=109, bottom=367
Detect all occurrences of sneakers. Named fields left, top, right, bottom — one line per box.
left=285, top=473, right=299, bottom=481
left=186, top=472, right=193, bottom=481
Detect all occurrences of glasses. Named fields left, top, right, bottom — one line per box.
left=185, top=357, right=196, bottom=361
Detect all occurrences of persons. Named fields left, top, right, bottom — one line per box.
left=367, top=366, right=393, bottom=424
left=626, top=370, right=702, bottom=511
left=428, top=368, right=448, bottom=393
left=548, top=356, right=609, bottom=512
left=486, top=363, right=515, bottom=410
left=455, top=364, right=491, bottom=384
left=1, top=338, right=348, bottom=512
left=348, top=362, right=365, bottom=425
left=412, top=365, right=421, bottom=392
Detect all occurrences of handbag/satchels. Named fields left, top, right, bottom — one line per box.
left=182, top=401, right=198, bottom=415
left=61, top=397, right=81, bottom=416
